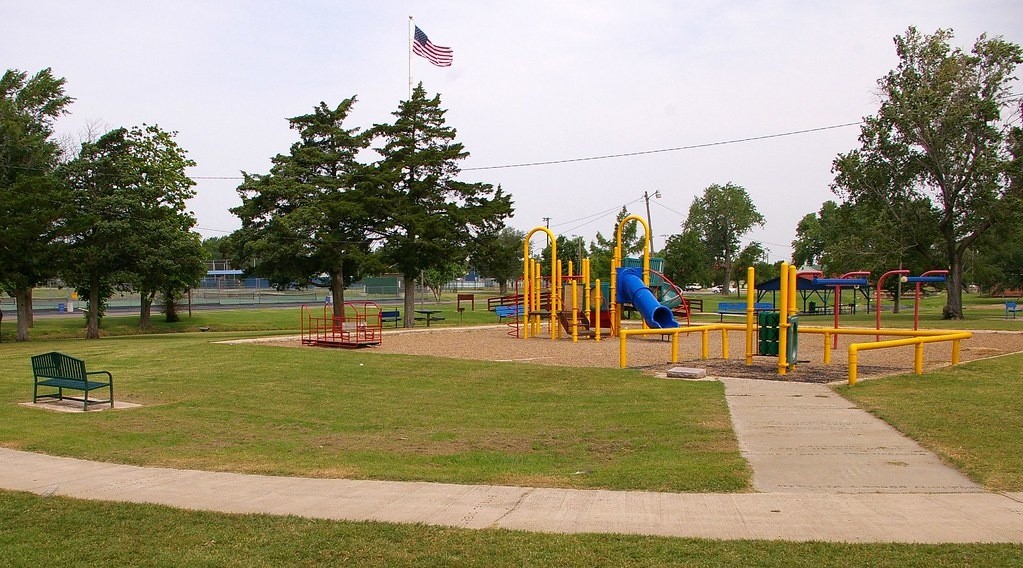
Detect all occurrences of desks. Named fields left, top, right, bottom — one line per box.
left=414, top=310, right=442, bottom=327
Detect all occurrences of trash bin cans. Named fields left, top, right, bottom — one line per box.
left=809, top=301, right=816, bottom=312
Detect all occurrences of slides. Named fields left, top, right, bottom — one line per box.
left=624, top=275, right=678, bottom=336
left=656, top=282, right=684, bottom=309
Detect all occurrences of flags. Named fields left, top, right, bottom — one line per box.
left=412, top=25, right=453, bottom=67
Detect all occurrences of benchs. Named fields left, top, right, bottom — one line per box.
left=718, top=303, right=773, bottom=322
left=495, top=305, right=524, bottom=322
left=1006, top=301, right=1022, bottom=320
left=414, top=317, right=447, bottom=321
left=379, top=308, right=402, bottom=327
left=30, top=351, right=114, bottom=410
